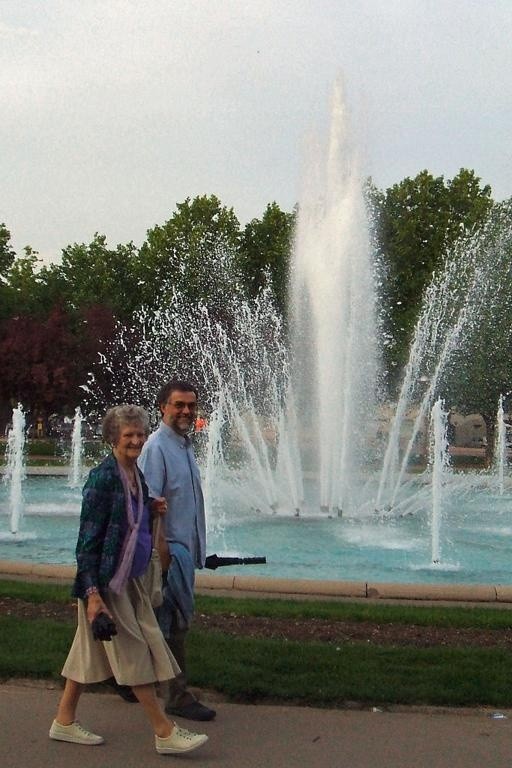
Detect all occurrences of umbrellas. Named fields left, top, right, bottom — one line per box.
left=89, top=612, right=117, bottom=644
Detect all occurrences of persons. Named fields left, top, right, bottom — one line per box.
left=102, top=381, right=216, bottom=721
left=49, top=405, right=209, bottom=755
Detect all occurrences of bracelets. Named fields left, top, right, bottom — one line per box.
left=84, top=584, right=99, bottom=597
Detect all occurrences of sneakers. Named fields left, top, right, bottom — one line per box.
left=165, top=693, right=216, bottom=720
left=105, top=675, right=139, bottom=704
left=49, top=719, right=103, bottom=745
left=155, top=720, right=208, bottom=754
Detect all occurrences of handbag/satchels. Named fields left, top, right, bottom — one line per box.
left=142, top=547, right=164, bottom=607
